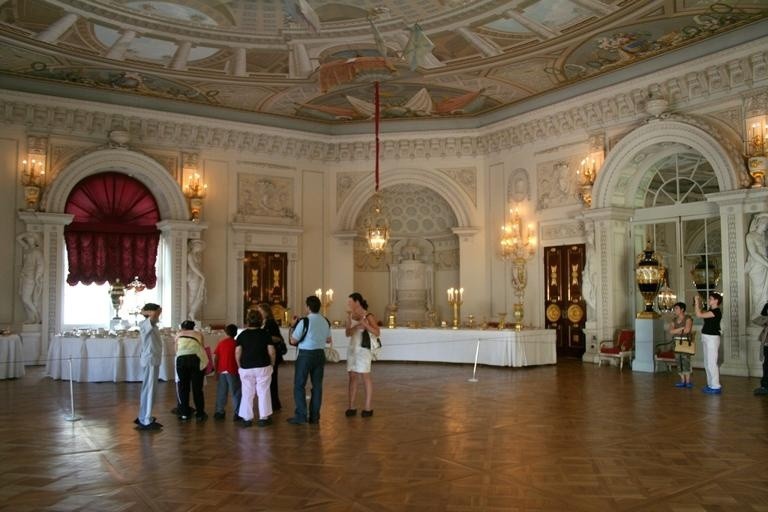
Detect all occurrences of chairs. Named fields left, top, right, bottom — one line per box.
left=598, top=328, right=634, bottom=371
left=655, top=337, right=684, bottom=376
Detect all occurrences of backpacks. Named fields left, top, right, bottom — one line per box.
left=289, top=317, right=331, bottom=346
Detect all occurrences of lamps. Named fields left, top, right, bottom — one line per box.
left=742, top=122, right=768, bottom=188
left=21, top=159, right=46, bottom=212
left=365, top=82, right=389, bottom=260
left=313, top=288, right=335, bottom=317
left=575, top=155, right=596, bottom=208
left=499, top=207, right=535, bottom=260
left=182, top=172, right=208, bottom=222
left=446, top=287, right=464, bottom=329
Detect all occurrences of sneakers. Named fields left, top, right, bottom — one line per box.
left=675, top=382, right=722, bottom=394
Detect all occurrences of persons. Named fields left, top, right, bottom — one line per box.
left=185, top=238, right=208, bottom=322
left=212, top=322, right=242, bottom=422
left=744, top=213, right=768, bottom=326
left=133, top=302, right=164, bottom=431
left=234, top=308, right=277, bottom=428
left=693, top=291, right=728, bottom=394
left=285, top=294, right=333, bottom=428
left=13, top=230, right=43, bottom=327
left=751, top=299, right=767, bottom=397
left=577, top=220, right=597, bottom=325
left=173, top=320, right=209, bottom=425
left=668, top=302, right=697, bottom=389
left=257, top=303, right=287, bottom=416
left=343, top=291, right=381, bottom=415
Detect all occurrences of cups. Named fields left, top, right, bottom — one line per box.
left=60, top=327, right=212, bottom=337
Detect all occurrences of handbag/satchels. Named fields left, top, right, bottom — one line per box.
left=203, top=346, right=214, bottom=374
left=360, top=313, right=383, bottom=349
left=673, top=329, right=696, bottom=355
left=324, top=343, right=340, bottom=363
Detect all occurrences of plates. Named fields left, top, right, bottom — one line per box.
left=440, top=325, right=448, bottom=328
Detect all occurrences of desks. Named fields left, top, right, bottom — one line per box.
left=1, top=323, right=27, bottom=384
left=49, top=326, right=226, bottom=383
left=238, top=317, right=558, bottom=369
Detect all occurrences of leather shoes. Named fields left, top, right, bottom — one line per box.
left=361, top=410, right=372, bottom=417
left=287, top=416, right=307, bottom=424
left=345, top=409, right=356, bottom=417
left=136, top=406, right=271, bottom=432
